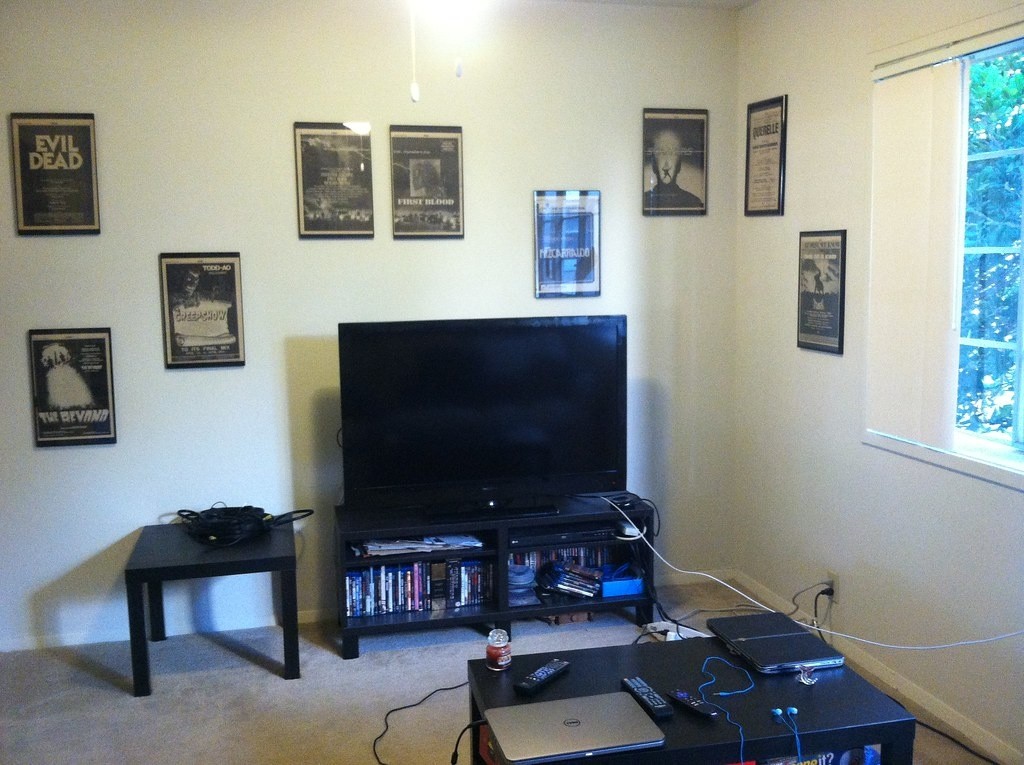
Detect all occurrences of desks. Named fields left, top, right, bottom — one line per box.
left=464, top=633, right=917, bottom=765
left=124, top=514, right=299, bottom=696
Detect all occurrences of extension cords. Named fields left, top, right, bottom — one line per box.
left=645, top=622, right=711, bottom=642
left=616, top=520, right=639, bottom=536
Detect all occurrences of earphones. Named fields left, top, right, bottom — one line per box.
left=772, top=708, right=782, bottom=715
left=787, top=707, right=798, bottom=715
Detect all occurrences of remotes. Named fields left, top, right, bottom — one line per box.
left=666, top=689, right=718, bottom=717
left=512, top=658, right=571, bottom=696
left=622, top=676, right=674, bottom=717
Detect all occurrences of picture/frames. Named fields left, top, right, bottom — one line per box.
left=27, top=328, right=117, bottom=448
left=796, top=229, right=847, bottom=354
left=293, top=121, right=375, bottom=238
left=10, top=112, right=100, bottom=235
left=743, top=95, right=789, bottom=215
left=157, top=253, right=246, bottom=369
left=389, top=124, right=464, bottom=238
left=640, top=108, right=708, bottom=215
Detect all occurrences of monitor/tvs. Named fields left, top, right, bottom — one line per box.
left=338, top=315, right=628, bottom=525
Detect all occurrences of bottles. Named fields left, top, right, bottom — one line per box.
left=485, top=628, right=512, bottom=671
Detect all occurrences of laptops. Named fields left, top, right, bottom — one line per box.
left=482, top=691, right=665, bottom=765
left=707, top=612, right=845, bottom=673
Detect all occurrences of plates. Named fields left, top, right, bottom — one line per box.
left=507, top=572, right=534, bottom=586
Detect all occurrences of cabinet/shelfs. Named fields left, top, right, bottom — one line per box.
left=333, top=492, right=657, bottom=660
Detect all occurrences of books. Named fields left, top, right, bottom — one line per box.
left=508, top=546, right=623, bottom=598
left=345, top=559, right=493, bottom=616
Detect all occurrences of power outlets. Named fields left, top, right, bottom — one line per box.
left=827, top=567, right=839, bottom=602
